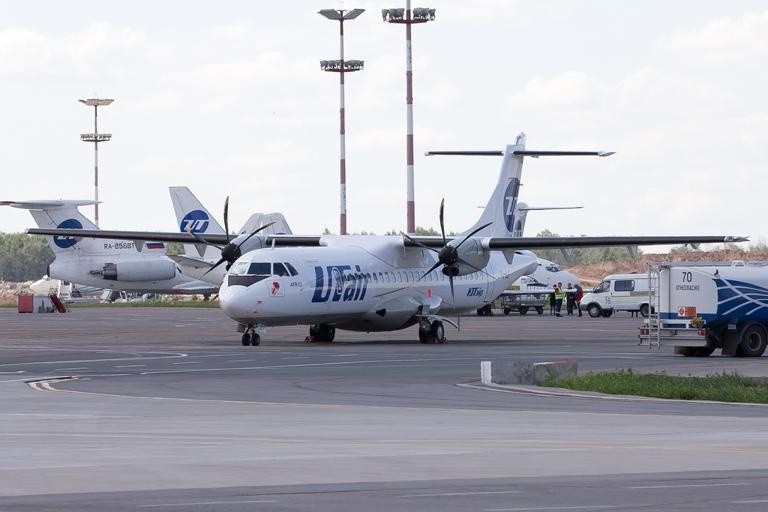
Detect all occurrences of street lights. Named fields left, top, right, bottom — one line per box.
left=317, top=8, right=366, bottom=237
left=78, top=96, right=115, bottom=229
left=381, top=0, right=436, bottom=234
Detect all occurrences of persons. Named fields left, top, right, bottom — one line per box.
left=572, top=284, right=583, bottom=317
left=553, top=282, right=565, bottom=317
left=563, top=283, right=575, bottom=316
left=549, top=284, right=556, bottom=315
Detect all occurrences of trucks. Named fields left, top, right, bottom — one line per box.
left=639, top=259, right=768, bottom=358
